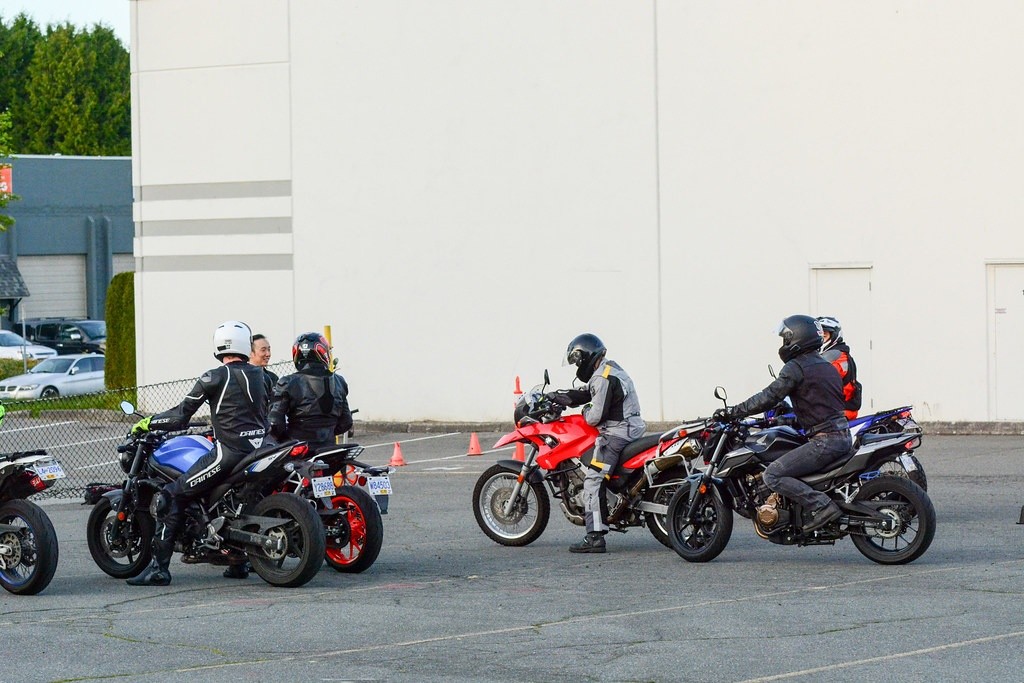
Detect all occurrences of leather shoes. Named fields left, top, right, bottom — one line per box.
left=802, top=502, right=843, bottom=533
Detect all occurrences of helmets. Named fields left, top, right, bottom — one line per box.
left=778, top=315, right=824, bottom=364
left=815, top=316, right=841, bottom=355
left=561, top=333, right=607, bottom=383
left=292, top=332, right=334, bottom=373
left=213, top=320, right=254, bottom=361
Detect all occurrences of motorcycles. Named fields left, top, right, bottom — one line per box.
left=81, top=401, right=394, bottom=588
left=742, top=365, right=928, bottom=520
left=667, top=386, right=937, bottom=565
left=0, top=448, right=67, bottom=595
left=472, top=369, right=716, bottom=549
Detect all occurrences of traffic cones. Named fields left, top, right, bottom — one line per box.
left=466, top=431, right=484, bottom=456
left=389, top=442, right=407, bottom=467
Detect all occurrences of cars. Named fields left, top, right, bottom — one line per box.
left=0, top=354, right=106, bottom=404
left=0, top=329, right=58, bottom=360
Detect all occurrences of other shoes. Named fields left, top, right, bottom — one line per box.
left=569, top=536, right=607, bottom=553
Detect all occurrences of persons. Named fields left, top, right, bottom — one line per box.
left=124, top=321, right=272, bottom=586
left=545, top=332, right=648, bottom=553
left=714, top=315, right=854, bottom=538
left=247, top=332, right=354, bottom=573
left=819, top=316, right=864, bottom=422
left=248, top=334, right=280, bottom=387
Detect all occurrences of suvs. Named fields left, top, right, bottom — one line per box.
left=11, top=317, right=107, bottom=354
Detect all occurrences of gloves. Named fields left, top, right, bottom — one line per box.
left=713, top=408, right=734, bottom=424
left=580, top=403, right=593, bottom=416
left=538, top=392, right=551, bottom=410
left=131, top=415, right=153, bottom=435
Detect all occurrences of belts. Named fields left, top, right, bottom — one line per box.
left=815, top=431, right=850, bottom=437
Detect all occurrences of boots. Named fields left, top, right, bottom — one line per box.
left=223, top=562, right=249, bottom=579
left=125, top=536, right=177, bottom=586
